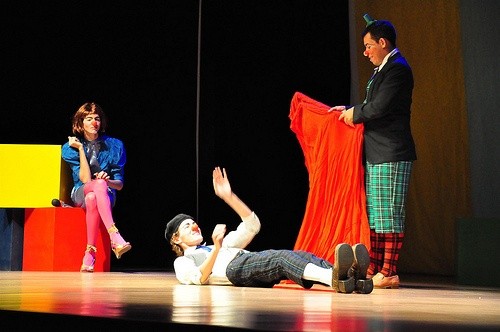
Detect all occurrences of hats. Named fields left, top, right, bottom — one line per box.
left=165, top=214, right=192, bottom=240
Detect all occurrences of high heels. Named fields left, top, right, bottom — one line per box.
left=108, top=227, right=132, bottom=259
left=79, top=245, right=98, bottom=272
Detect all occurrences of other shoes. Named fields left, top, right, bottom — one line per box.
left=371, top=272, right=400, bottom=289
left=332, top=243, right=356, bottom=294
left=352, top=244, right=374, bottom=294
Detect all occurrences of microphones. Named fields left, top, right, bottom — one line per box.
left=52, top=199, right=70, bottom=207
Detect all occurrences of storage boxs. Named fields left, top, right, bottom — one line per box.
left=0, top=144, right=75, bottom=209
left=22, top=208, right=112, bottom=272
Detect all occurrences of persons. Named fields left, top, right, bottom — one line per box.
left=62, top=102, right=132, bottom=273
left=327, top=20, right=415, bottom=289
left=165, top=166, right=374, bottom=294
